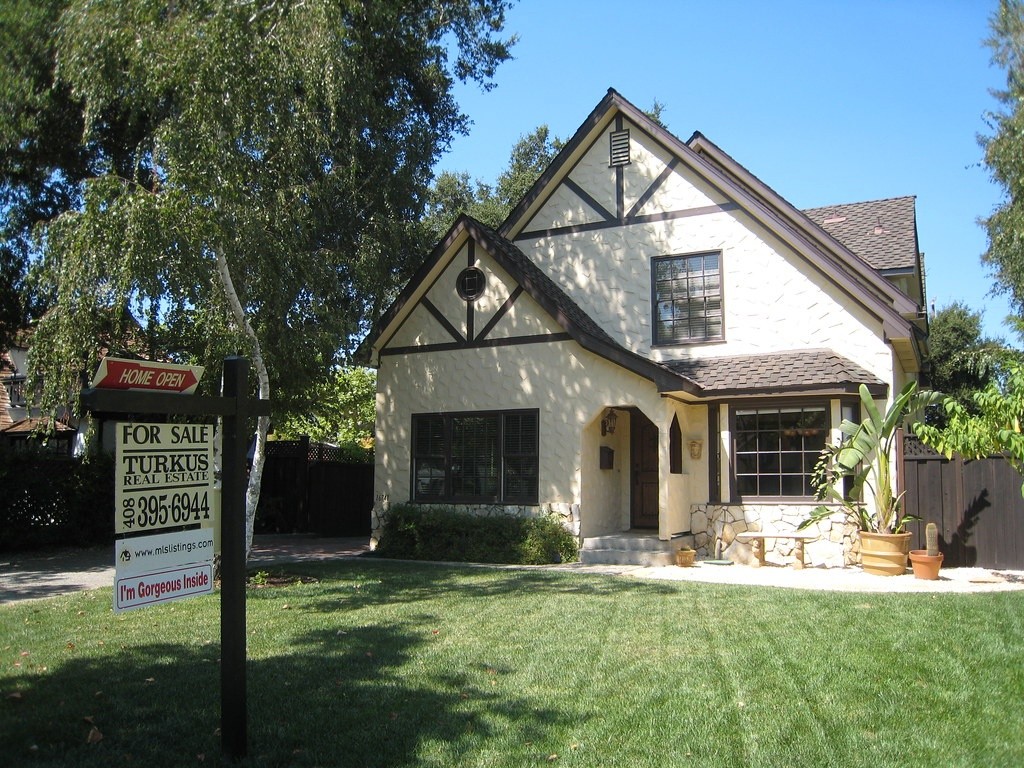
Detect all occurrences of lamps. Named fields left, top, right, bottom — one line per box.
left=601, top=408, right=618, bottom=436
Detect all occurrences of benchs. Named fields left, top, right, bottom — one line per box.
left=737, top=530, right=819, bottom=570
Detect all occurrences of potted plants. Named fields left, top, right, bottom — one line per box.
left=676, top=545, right=696, bottom=567
left=796, top=380, right=925, bottom=576
left=909, top=523, right=944, bottom=580
